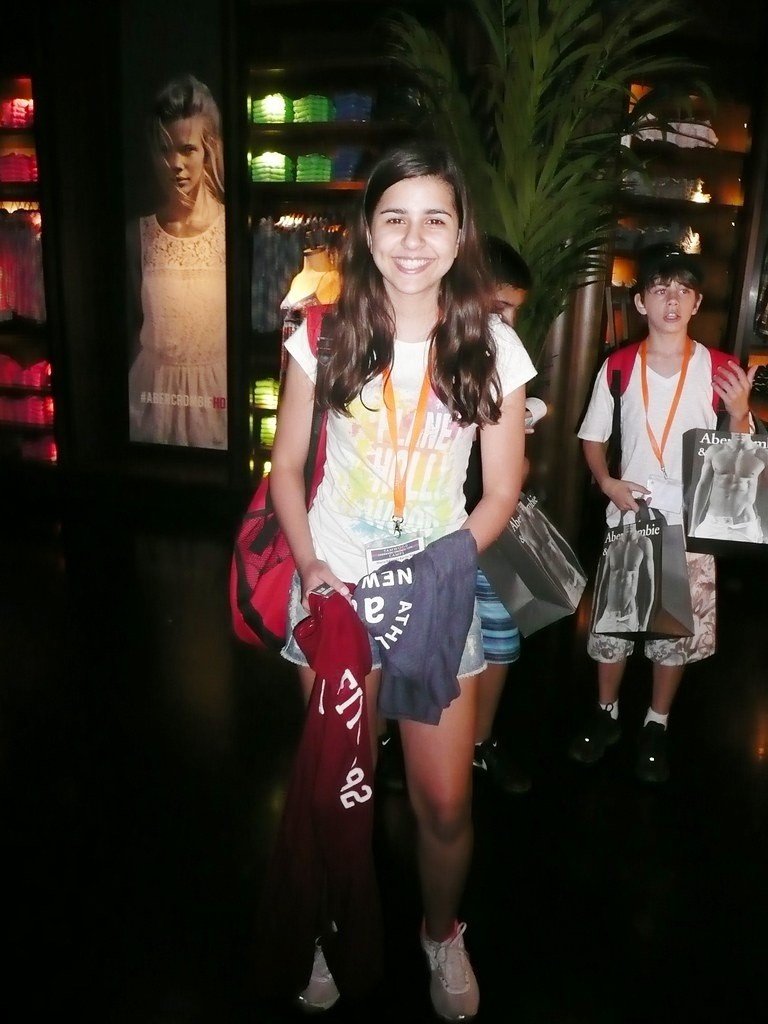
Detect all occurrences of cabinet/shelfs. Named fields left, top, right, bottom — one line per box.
left=0, top=0, right=768, bottom=519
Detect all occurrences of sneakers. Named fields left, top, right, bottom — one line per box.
left=375, top=732, right=394, bottom=763
left=473, top=735, right=533, bottom=794
left=419, top=915, right=479, bottom=1019
left=634, top=722, right=672, bottom=784
left=294, top=937, right=340, bottom=1010
left=570, top=703, right=622, bottom=762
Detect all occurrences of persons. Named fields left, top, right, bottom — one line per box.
left=375, top=233, right=536, bottom=792
left=568, top=245, right=759, bottom=783
left=688, top=431, right=768, bottom=545
left=592, top=524, right=655, bottom=632
left=514, top=498, right=588, bottom=607
left=124, top=72, right=229, bottom=450
left=278, top=141, right=541, bottom=1024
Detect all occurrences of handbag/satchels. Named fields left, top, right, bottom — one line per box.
left=475, top=490, right=588, bottom=640
left=681, top=406, right=768, bottom=560
left=588, top=499, right=695, bottom=638
left=230, top=468, right=297, bottom=650
